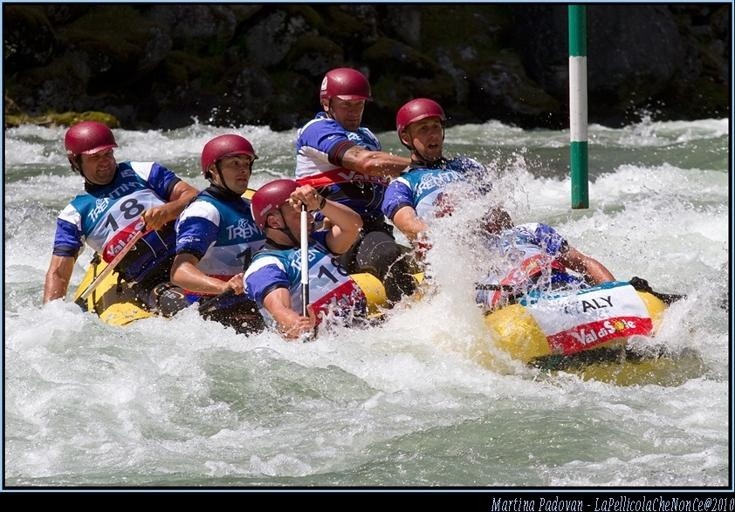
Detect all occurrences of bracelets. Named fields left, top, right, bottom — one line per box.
left=315, top=196, right=326, bottom=213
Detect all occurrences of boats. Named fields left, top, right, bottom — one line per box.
left=71, top=179, right=671, bottom=371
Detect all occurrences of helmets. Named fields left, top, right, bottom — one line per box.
left=396, top=99, right=446, bottom=131
left=250, top=179, right=300, bottom=229
left=65, top=120, right=119, bottom=155
left=320, top=67, right=372, bottom=105
left=202, top=134, right=258, bottom=175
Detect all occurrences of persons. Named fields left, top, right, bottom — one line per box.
left=42, top=120, right=200, bottom=319
left=170, top=134, right=265, bottom=338
left=295, top=67, right=417, bottom=308
left=473, top=206, right=615, bottom=311
left=381, top=98, right=493, bottom=302
left=243, top=179, right=381, bottom=341
